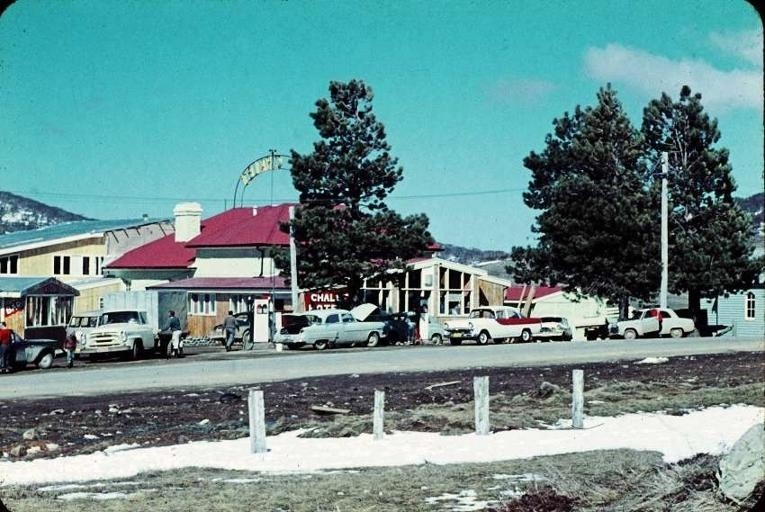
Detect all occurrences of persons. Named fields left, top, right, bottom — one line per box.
left=410, top=308, right=421, bottom=340
left=221, top=310, right=239, bottom=351
left=650, top=305, right=662, bottom=338
left=0, top=322, right=14, bottom=374
left=62, top=330, right=76, bottom=368
left=151, top=311, right=186, bottom=358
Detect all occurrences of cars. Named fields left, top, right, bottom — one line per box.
left=5, top=309, right=162, bottom=368
left=610, top=306, right=697, bottom=340
left=210, top=309, right=253, bottom=346
left=407, top=305, right=573, bottom=346
left=272, top=302, right=389, bottom=350
